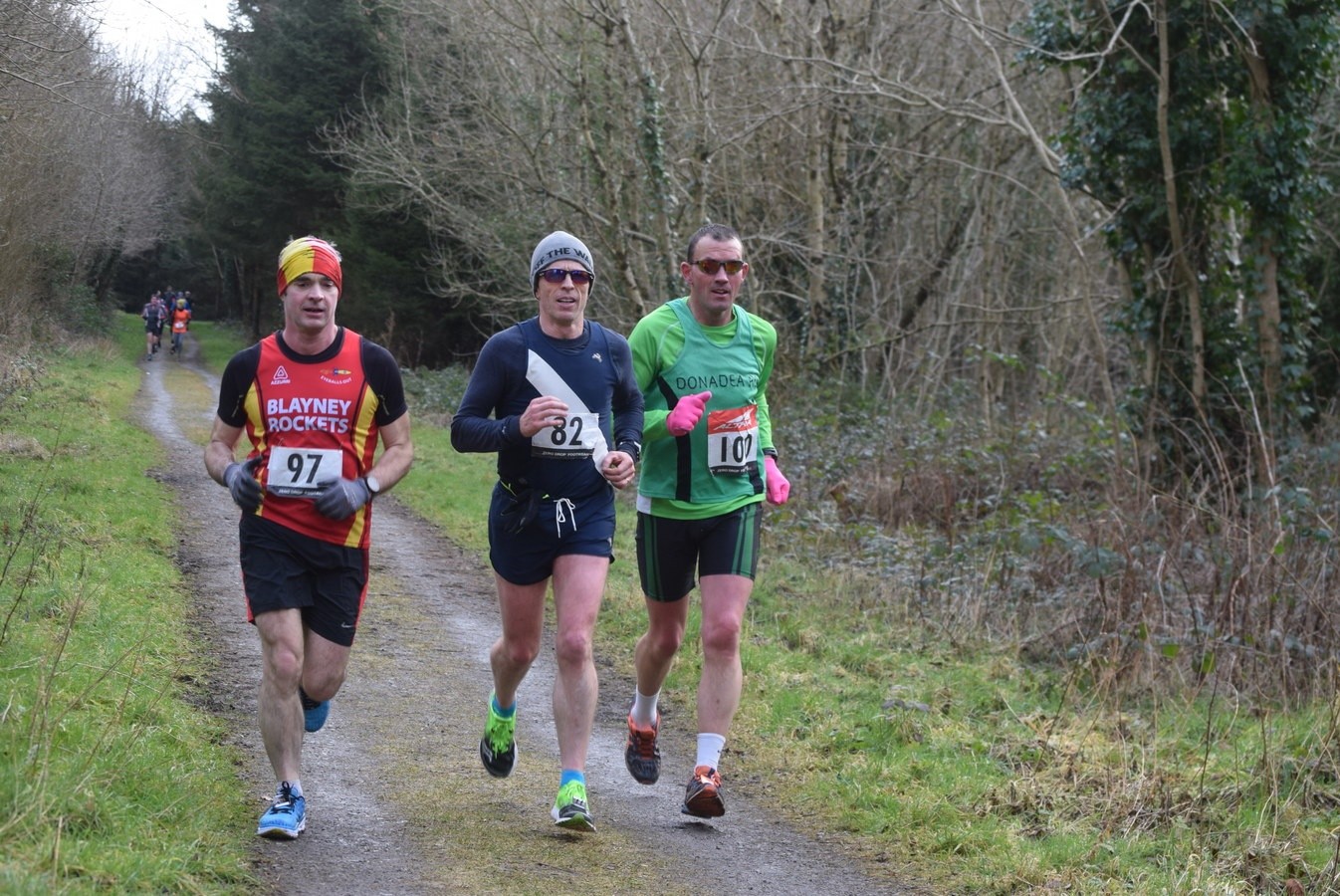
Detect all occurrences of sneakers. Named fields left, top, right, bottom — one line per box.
left=680, top=764, right=726, bottom=818
left=479, top=687, right=518, bottom=778
left=297, top=684, right=330, bottom=732
left=625, top=709, right=661, bottom=785
left=257, top=780, right=306, bottom=840
left=551, top=779, right=597, bottom=833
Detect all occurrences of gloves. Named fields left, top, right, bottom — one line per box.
left=314, top=476, right=371, bottom=521
left=223, top=456, right=264, bottom=514
left=764, top=458, right=791, bottom=505
left=666, top=391, right=712, bottom=436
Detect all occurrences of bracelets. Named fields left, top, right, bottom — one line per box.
left=761, top=447, right=779, bottom=463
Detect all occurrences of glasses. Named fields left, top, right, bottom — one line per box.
left=690, top=259, right=743, bottom=275
left=537, top=268, right=594, bottom=285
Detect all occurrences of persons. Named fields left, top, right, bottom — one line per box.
left=449, top=230, right=647, bottom=836
left=202, top=230, right=415, bottom=841
left=612, top=224, right=792, bottom=823
left=142, top=282, right=193, bottom=364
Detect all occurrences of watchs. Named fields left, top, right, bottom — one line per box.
left=362, top=475, right=381, bottom=502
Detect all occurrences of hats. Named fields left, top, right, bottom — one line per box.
left=276, top=236, right=343, bottom=301
left=529, top=231, right=595, bottom=301
